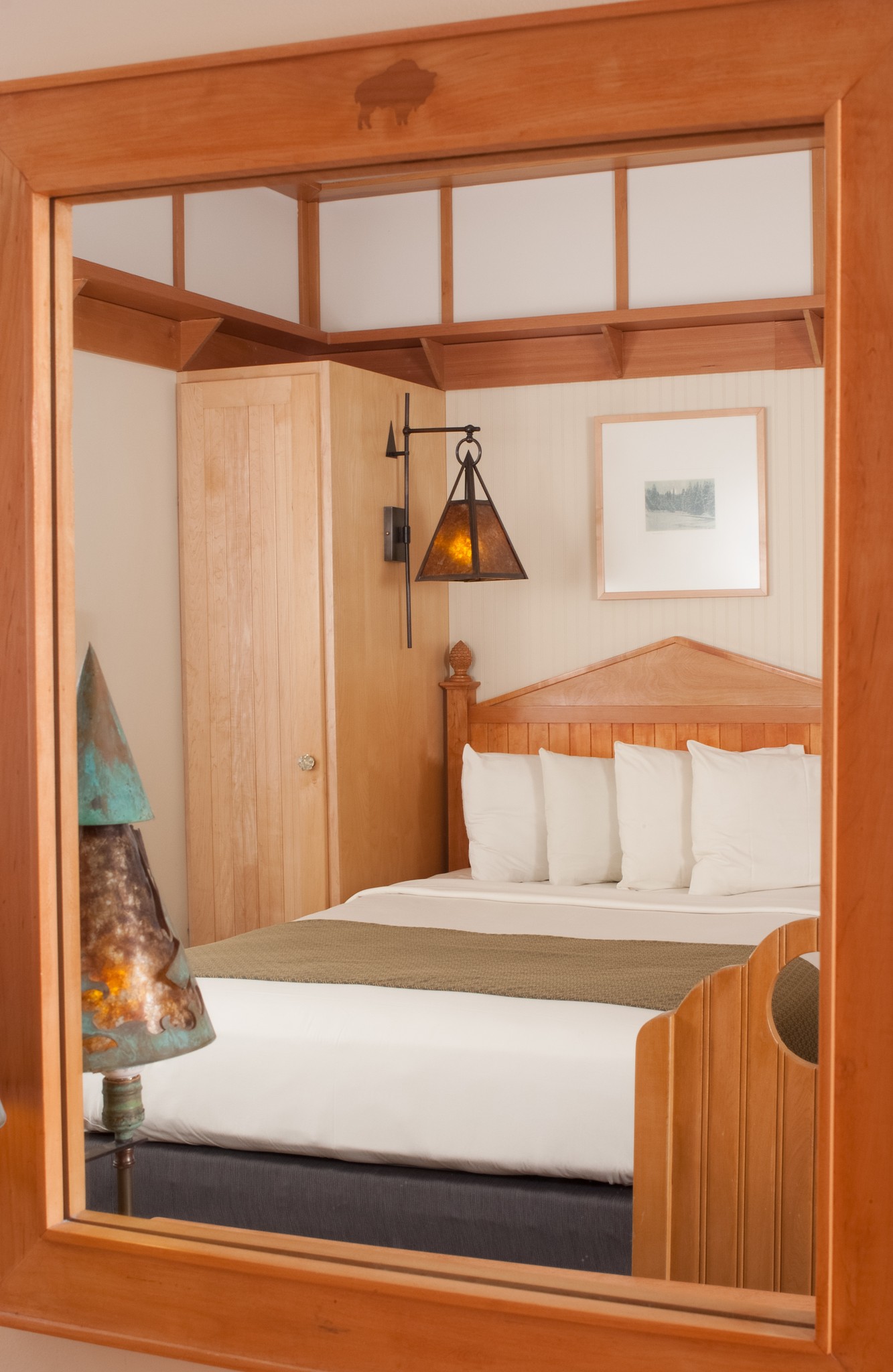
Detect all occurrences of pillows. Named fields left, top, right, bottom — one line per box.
left=457, top=737, right=823, bottom=903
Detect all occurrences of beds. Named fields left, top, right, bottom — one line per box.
left=78, top=634, right=825, bottom=1284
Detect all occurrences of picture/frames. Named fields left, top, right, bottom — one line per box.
left=592, top=404, right=771, bottom=602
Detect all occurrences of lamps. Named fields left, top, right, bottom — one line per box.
left=75, top=640, right=220, bottom=1219
left=381, top=392, right=530, bottom=648
